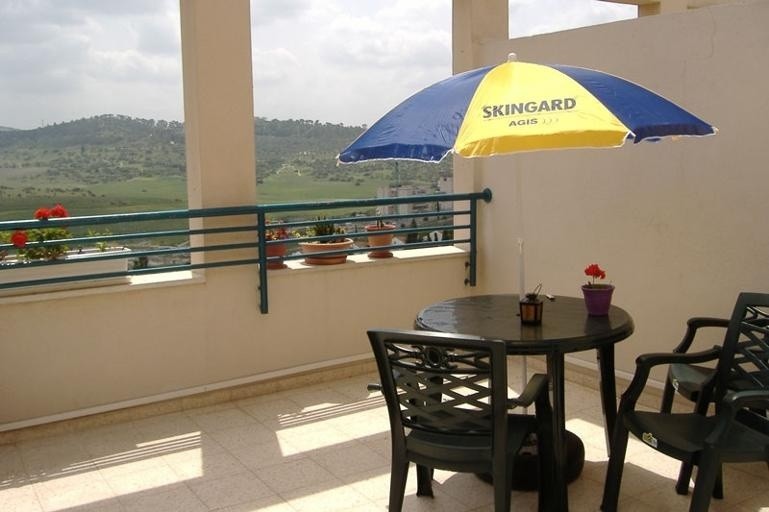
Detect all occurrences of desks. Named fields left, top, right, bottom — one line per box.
left=414, top=294, right=634, bottom=512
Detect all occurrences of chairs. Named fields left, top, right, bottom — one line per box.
left=366, top=330, right=554, bottom=512
left=660, top=317, right=769, bottom=499
left=598, top=295, right=769, bottom=511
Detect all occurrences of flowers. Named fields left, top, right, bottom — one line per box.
left=583, top=262, right=607, bottom=285
left=9, top=204, right=72, bottom=262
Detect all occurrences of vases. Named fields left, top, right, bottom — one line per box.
left=0, top=246, right=132, bottom=298
left=581, top=285, right=615, bottom=316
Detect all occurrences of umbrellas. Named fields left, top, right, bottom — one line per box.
left=336, top=52, right=718, bottom=415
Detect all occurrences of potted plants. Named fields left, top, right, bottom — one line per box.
left=259, top=212, right=396, bottom=269
